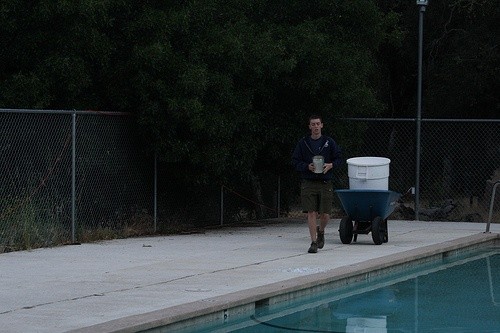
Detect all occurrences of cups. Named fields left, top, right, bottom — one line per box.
left=313, top=156, right=324, bottom=173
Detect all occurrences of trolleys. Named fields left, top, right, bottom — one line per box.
left=333, top=187, right=415, bottom=245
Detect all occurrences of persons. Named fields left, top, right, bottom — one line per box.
left=292, top=115, right=343, bottom=254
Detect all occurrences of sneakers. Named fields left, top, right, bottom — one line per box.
left=316, top=226, right=325, bottom=249
left=308, top=241, right=317, bottom=253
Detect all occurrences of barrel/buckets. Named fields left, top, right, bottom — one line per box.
left=346, top=157, right=390, bottom=191
